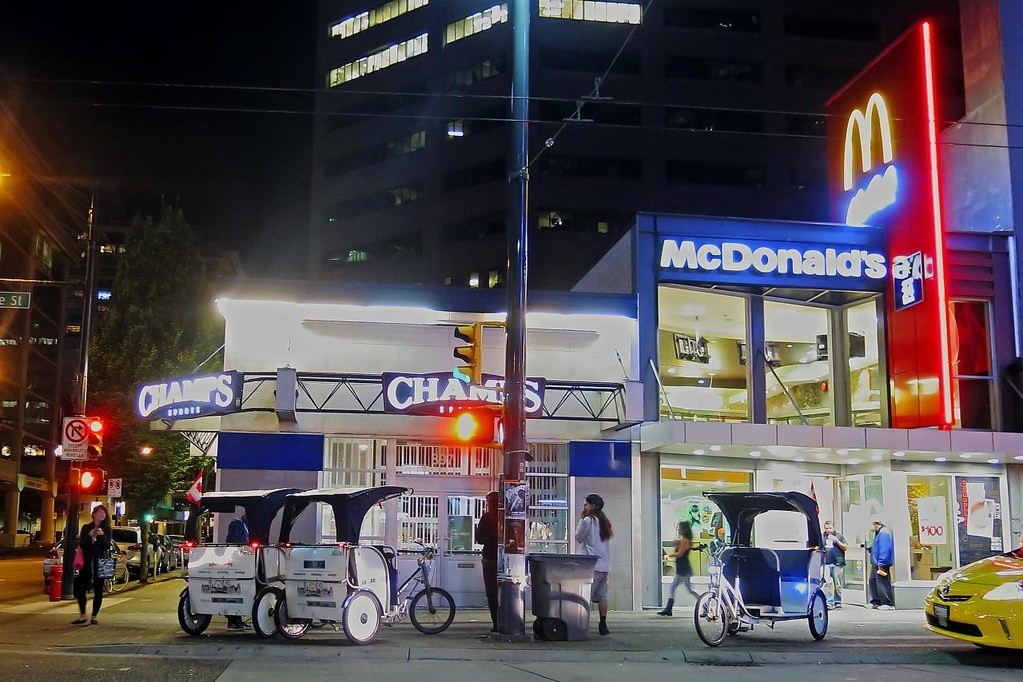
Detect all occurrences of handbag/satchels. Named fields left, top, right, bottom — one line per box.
left=73, top=546, right=84, bottom=570
left=96, top=542, right=118, bottom=579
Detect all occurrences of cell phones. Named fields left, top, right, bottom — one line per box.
left=96, top=529, right=101, bottom=531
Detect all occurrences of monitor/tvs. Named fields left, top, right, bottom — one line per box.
left=816, top=334, right=866, bottom=361
left=735, top=340, right=781, bottom=367
left=673, top=334, right=710, bottom=364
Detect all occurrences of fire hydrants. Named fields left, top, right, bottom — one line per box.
left=43, top=561, right=63, bottom=602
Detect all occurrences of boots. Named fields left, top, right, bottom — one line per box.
left=657, top=597, right=674, bottom=616
left=599, top=616, right=610, bottom=635
left=699, top=601, right=712, bottom=617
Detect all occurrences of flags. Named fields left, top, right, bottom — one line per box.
left=186, top=470, right=202, bottom=506
left=810, top=481, right=819, bottom=514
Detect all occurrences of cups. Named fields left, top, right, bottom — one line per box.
left=877, top=570, right=887, bottom=577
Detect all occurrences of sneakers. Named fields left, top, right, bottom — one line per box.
left=227, top=621, right=245, bottom=631
left=864, top=603, right=879, bottom=609
left=240, top=621, right=253, bottom=630
left=877, top=604, right=897, bottom=609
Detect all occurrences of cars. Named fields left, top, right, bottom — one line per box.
left=43, top=526, right=195, bottom=586
left=923, top=547, right=1023, bottom=650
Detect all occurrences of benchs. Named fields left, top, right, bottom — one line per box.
left=771, top=549, right=822, bottom=587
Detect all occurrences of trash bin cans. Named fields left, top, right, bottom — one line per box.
left=529, top=553, right=601, bottom=642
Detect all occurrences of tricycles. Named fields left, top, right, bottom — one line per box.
left=689, top=491, right=828, bottom=647
left=178, top=488, right=328, bottom=639
left=274, top=485, right=456, bottom=645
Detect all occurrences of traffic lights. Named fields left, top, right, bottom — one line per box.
left=453, top=408, right=495, bottom=445
left=79, top=468, right=104, bottom=494
left=143, top=512, right=155, bottom=524
left=87, top=420, right=104, bottom=457
left=454, top=322, right=482, bottom=386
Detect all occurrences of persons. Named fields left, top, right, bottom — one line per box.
left=518, top=489, right=525, bottom=511
left=822, top=521, right=849, bottom=610
left=476, top=492, right=497, bottom=631
left=70, top=505, right=111, bottom=624
left=710, top=528, right=724, bottom=565
left=864, top=519, right=896, bottom=609
left=225, top=514, right=252, bottom=631
left=657, top=522, right=708, bottom=618
left=576, top=494, right=612, bottom=634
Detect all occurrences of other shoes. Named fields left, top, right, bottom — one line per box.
left=91, top=619, right=97, bottom=624
left=827, top=603, right=836, bottom=610
left=834, top=602, right=842, bottom=608
left=70, top=618, right=87, bottom=625
